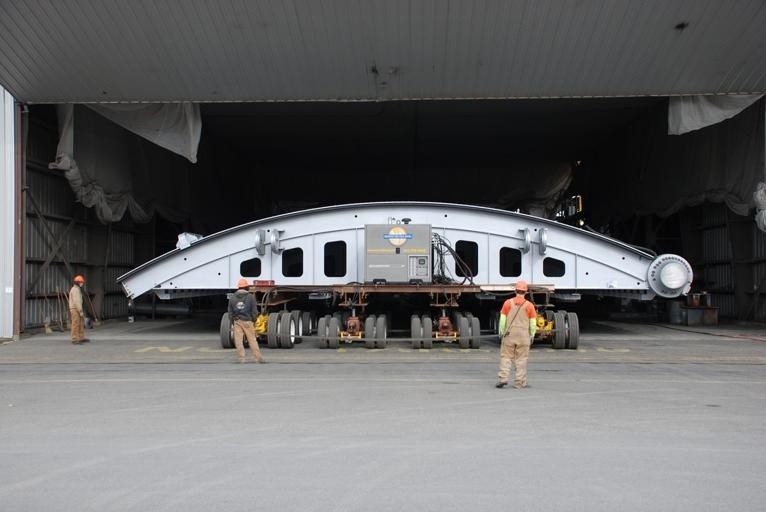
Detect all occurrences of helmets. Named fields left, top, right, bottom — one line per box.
left=237, top=278, right=249, bottom=288
left=515, top=281, right=528, bottom=292
left=74, top=275, right=84, bottom=282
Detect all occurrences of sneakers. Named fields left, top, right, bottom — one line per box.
left=495, top=381, right=507, bottom=388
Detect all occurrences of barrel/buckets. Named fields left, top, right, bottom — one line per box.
left=669, top=301, right=684, bottom=324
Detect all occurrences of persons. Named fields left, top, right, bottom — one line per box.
left=227, top=279, right=268, bottom=364
left=67, top=274, right=91, bottom=345
left=494, top=281, right=538, bottom=389
